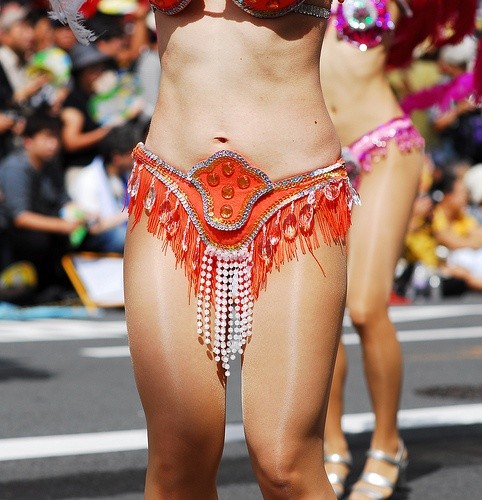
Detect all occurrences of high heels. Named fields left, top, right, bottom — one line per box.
left=324, top=450, right=356, bottom=498
left=347, top=438, right=411, bottom=500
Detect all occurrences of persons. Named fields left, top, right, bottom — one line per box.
left=317, top=0, right=438, bottom=500
left=42, top=0, right=349, bottom=500
left=0, top=0, right=482, bottom=306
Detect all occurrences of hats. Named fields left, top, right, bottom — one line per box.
left=70, top=42, right=109, bottom=71
